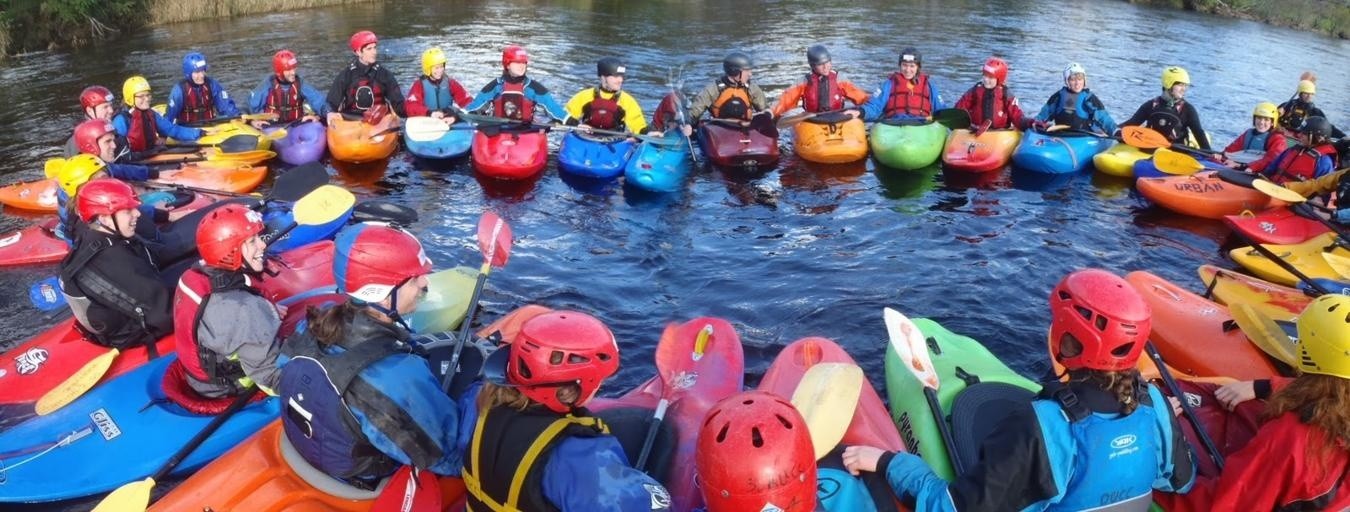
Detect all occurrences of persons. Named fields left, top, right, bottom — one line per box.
left=402, top=47, right=494, bottom=123
left=955, top=58, right=1047, bottom=132
left=688, top=391, right=898, bottom=512
left=768, top=45, right=871, bottom=121
left=46, top=53, right=236, bottom=352
left=840, top=267, right=1201, bottom=512
left=272, top=221, right=482, bottom=493
left=442, top=46, right=593, bottom=135
left=841, top=47, right=949, bottom=121
left=300, top=29, right=406, bottom=125
left=461, top=309, right=673, bottom=512
left=1147, top=293, right=1350, bottom=510
left=639, top=75, right=703, bottom=143
left=552, top=57, right=646, bottom=146
left=1116, top=66, right=1209, bottom=152
left=682, top=53, right=782, bottom=137
left=1209, top=79, right=1348, bottom=250
left=1032, top=61, right=1121, bottom=142
left=170, top=202, right=290, bottom=405
left=239, top=50, right=344, bottom=131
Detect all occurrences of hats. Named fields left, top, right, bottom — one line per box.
left=507, top=311, right=620, bottom=413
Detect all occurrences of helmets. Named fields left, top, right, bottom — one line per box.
left=332, top=220, right=433, bottom=303
left=78, top=85, right=112, bottom=109
left=350, top=30, right=379, bottom=53
left=1294, top=294, right=1350, bottom=378
left=1160, top=66, right=1191, bottom=90
left=1250, top=79, right=1334, bottom=139
left=182, top=52, right=207, bottom=79
left=502, top=44, right=528, bottom=68
left=77, top=179, right=142, bottom=223
left=1048, top=268, right=1153, bottom=370
left=697, top=392, right=817, bottom=512
left=121, top=75, right=152, bottom=106
left=807, top=45, right=833, bottom=68
left=982, top=57, right=1009, bottom=87
left=59, top=153, right=106, bottom=198
left=274, top=50, right=298, bottom=82
left=596, top=57, right=626, bottom=77
left=74, top=119, right=115, bottom=156
left=897, top=46, right=922, bottom=66
left=1064, top=61, right=1087, bottom=91
left=723, top=52, right=757, bottom=76
left=421, top=46, right=447, bottom=75
left=196, top=203, right=265, bottom=271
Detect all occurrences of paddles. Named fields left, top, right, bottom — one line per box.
left=458, top=112, right=589, bottom=131
left=249, top=160, right=330, bottom=211
left=1252, top=179, right=1349, bottom=220
left=1046, top=125, right=1071, bottom=133
left=368, top=125, right=405, bottom=139
left=407, top=113, right=452, bottom=142
left=165, top=134, right=258, bottom=153
left=549, top=127, right=677, bottom=145
left=777, top=107, right=857, bottom=128
left=790, top=363, right=864, bottom=461
left=369, top=209, right=512, bottom=512
left=1229, top=301, right=1304, bottom=379
left=885, top=307, right=965, bottom=478
left=1122, top=125, right=1217, bottom=158
left=179, top=112, right=280, bottom=124
left=1321, top=252, right=1350, bottom=279
left=35, top=185, right=356, bottom=417
left=45, top=149, right=270, bottom=178
left=92, top=382, right=259, bottom=511
left=970, top=119, right=992, bottom=137
left=1153, top=148, right=1262, bottom=175
left=634, top=322, right=697, bottom=475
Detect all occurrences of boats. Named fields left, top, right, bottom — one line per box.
left=1225, top=231, right=1349, bottom=293
left=884, top=318, right=1050, bottom=486
left=621, top=356, right=712, bottom=512
left=1196, top=264, right=1322, bottom=318
left=1123, top=268, right=1297, bottom=382
left=754, top=336, right=907, bottom=459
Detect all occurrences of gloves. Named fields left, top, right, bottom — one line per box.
left=1029, top=117, right=1045, bottom=130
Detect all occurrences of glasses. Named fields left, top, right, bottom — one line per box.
left=134, top=93, right=151, bottom=100
